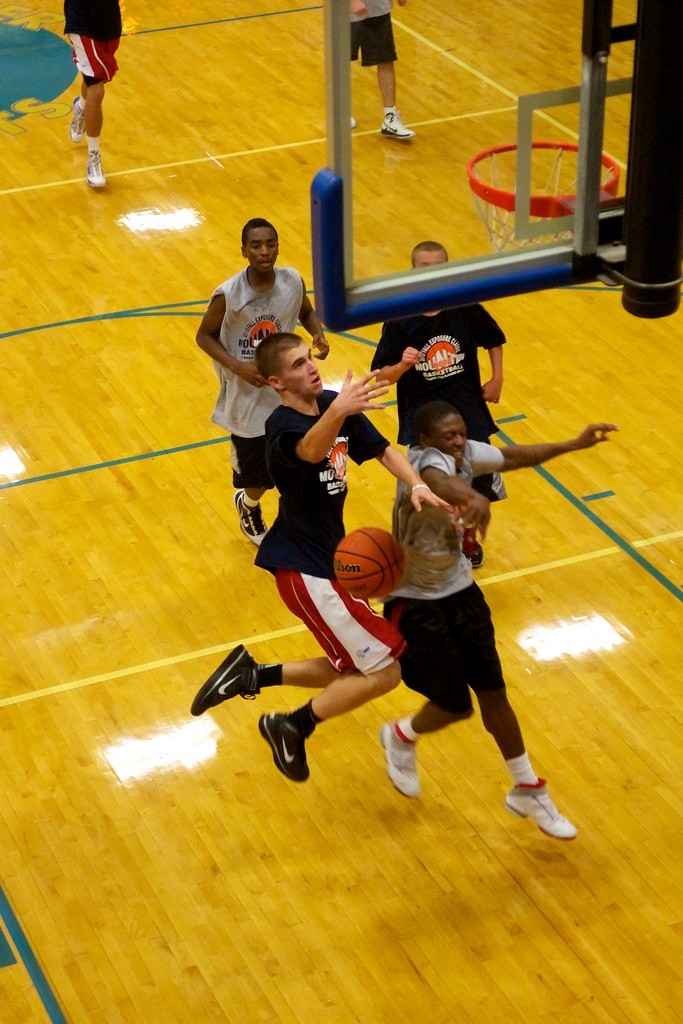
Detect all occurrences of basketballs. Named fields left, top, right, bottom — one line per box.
left=333, top=526, right=406, bottom=600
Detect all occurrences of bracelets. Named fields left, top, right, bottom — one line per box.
left=412, top=484, right=430, bottom=492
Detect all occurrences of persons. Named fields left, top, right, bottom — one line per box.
left=380, top=400, right=619, bottom=840
left=372, top=240, right=506, bottom=568
left=63, top=0, right=122, bottom=186
left=196, top=218, right=330, bottom=547
left=348, top=0, right=415, bottom=139
left=191, top=331, right=454, bottom=783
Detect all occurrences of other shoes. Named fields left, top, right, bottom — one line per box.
left=460, top=523, right=483, bottom=567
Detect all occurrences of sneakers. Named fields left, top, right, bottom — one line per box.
left=258, top=712, right=310, bottom=781
left=191, top=644, right=261, bottom=715
left=70, top=97, right=85, bottom=143
left=505, top=777, right=577, bottom=840
left=350, top=117, right=355, bottom=130
left=381, top=112, right=415, bottom=140
left=86, top=150, right=106, bottom=187
left=234, top=490, right=268, bottom=546
left=381, top=722, right=418, bottom=797
